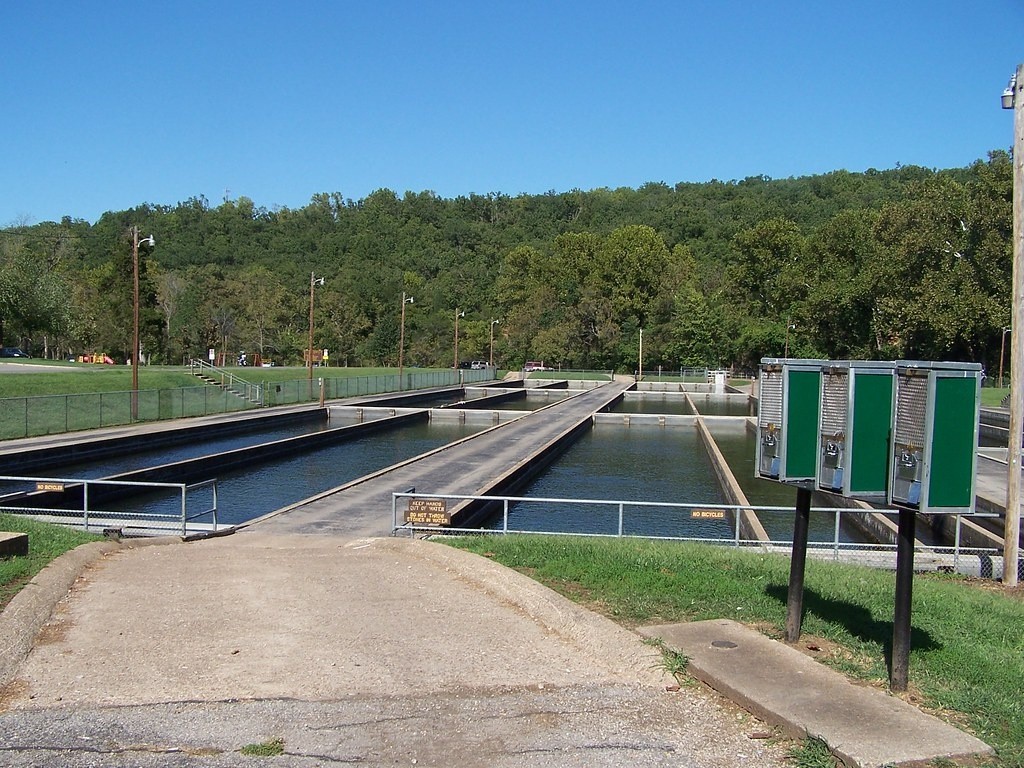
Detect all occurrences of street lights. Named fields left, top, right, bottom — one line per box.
left=400, top=293, right=413, bottom=392
left=1000, top=327, right=1012, bottom=389
left=785, top=320, right=795, bottom=359
left=455, top=308, right=464, bottom=369
left=131, top=227, right=155, bottom=420
left=491, top=317, right=499, bottom=369
left=308, top=271, right=325, bottom=400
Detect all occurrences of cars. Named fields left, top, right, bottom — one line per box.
left=65, top=352, right=87, bottom=361
left=0, top=348, right=32, bottom=358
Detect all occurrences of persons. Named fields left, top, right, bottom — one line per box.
left=238, top=350, right=246, bottom=363
left=83, top=348, right=87, bottom=356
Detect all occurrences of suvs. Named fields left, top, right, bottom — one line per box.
left=461, top=360, right=496, bottom=370
left=525, top=362, right=555, bottom=371
